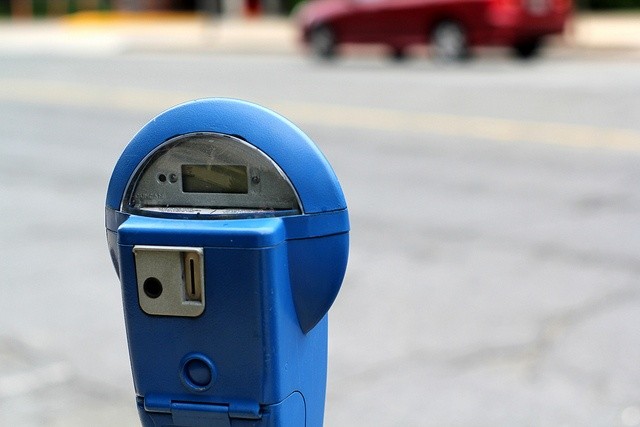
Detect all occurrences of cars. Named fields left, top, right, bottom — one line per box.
left=285, top=0, right=575, bottom=64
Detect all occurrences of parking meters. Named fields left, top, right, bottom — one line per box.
left=102, top=98, right=352, bottom=427
